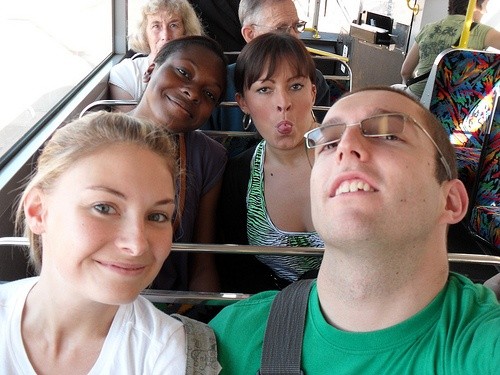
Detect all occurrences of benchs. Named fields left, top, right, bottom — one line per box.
left=0, top=237, right=500, bottom=305
left=128, top=52, right=352, bottom=94
left=81, top=101, right=331, bottom=143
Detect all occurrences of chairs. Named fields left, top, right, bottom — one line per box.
left=422, top=48, right=500, bottom=251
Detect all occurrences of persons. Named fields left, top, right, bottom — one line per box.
left=208, top=87, right=500, bottom=375
left=108, top=0, right=205, bottom=113
left=201, top=0, right=330, bottom=167
left=0, top=110, right=222, bottom=375
left=390, top=0, right=500, bottom=102
left=127, top=35, right=228, bottom=313
left=182, top=31, right=327, bottom=325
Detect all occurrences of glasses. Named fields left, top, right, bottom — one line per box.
left=250, top=20, right=307, bottom=33
left=304, top=112, right=452, bottom=181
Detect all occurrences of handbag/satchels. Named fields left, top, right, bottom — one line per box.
left=390, top=84, right=407, bottom=91
